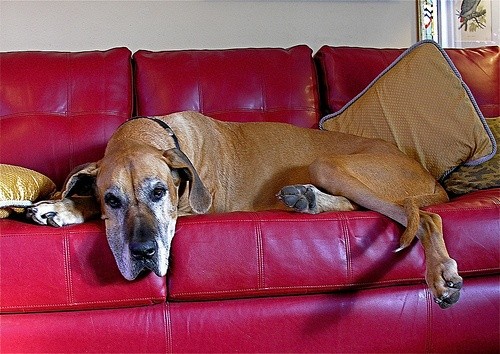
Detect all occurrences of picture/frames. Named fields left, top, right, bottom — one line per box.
left=418, top=0, right=500, bottom=48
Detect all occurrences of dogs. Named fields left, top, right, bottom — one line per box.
left=26, top=111, right=463, bottom=309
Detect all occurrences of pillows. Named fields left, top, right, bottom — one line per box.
left=319, top=40, right=497, bottom=188
left=442, top=117, right=500, bottom=194
left=1, top=163, right=56, bottom=218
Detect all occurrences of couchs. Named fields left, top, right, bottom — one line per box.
left=0, top=46, right=500, bottom=354
